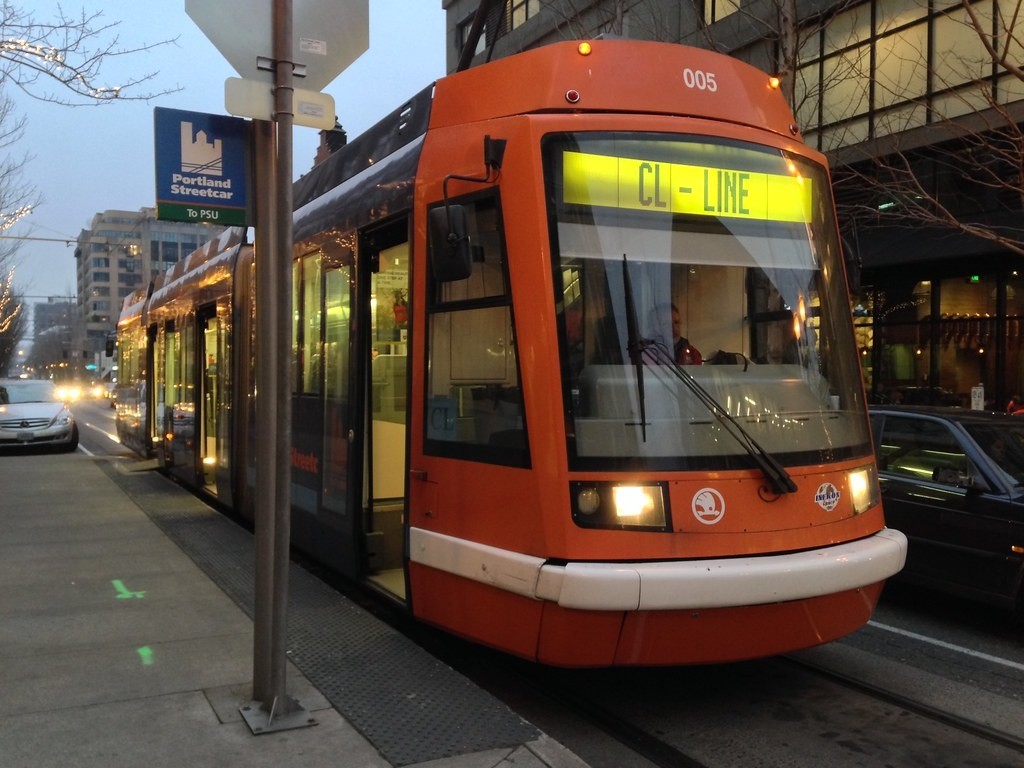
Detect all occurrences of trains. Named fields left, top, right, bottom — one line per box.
left=113, top=35, right=916, bottom=675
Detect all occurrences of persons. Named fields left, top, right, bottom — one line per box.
left=865, top=381, right=1024, bottom=469
left=389, top=289, right=408, bottom=343
left=634, top=301, right=703, bottom=366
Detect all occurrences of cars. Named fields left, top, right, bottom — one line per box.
left=864, top=403, right=1023, bottom=619
left=1, top=375, right=79, bottom=454
left=57, top=378, right=117, bottom=409
